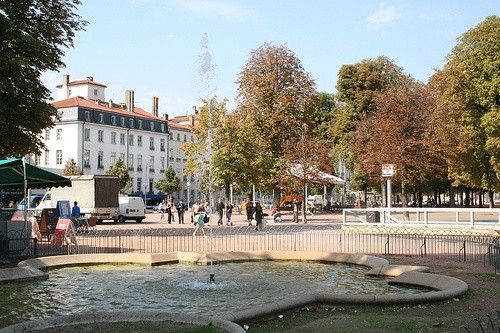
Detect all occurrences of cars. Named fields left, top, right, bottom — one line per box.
left=17, top=195, right=44, bottom=212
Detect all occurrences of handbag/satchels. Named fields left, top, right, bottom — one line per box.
left=193, top=215, right=200, bottom=222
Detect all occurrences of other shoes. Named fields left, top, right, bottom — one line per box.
left=193, top=232, right=196, bottom=237
left=204, top=235, right=207, bottom=239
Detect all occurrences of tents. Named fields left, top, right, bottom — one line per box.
left=280, top=164, right=344, bottom=185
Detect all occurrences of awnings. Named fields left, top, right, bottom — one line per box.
left=0, top=159, right=73, bottom=208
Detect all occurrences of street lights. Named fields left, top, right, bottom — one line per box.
left=186, top=182, right=190, bottom=212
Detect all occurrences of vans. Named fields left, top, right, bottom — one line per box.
left=118, top=196, right=146, bottom=223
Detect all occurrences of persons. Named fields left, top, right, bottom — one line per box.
left=191, top=199, right=212, bottom=238
left=158, top=198, right=176, bottom=222
left=176, top=199, right=185, bottom=224
left=246, top=202, right=262, bottom=231
left=216, top=198, right=225, bottom=226
left=226, top=201, right=235, bottom=226
left=271, top=206, right=284, bottom=222
left=71, top=201, right=80, bottom=220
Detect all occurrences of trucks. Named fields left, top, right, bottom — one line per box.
left=32, top=174, right=121, bottom=225
left=307, top=195, right=324, bottom=211
